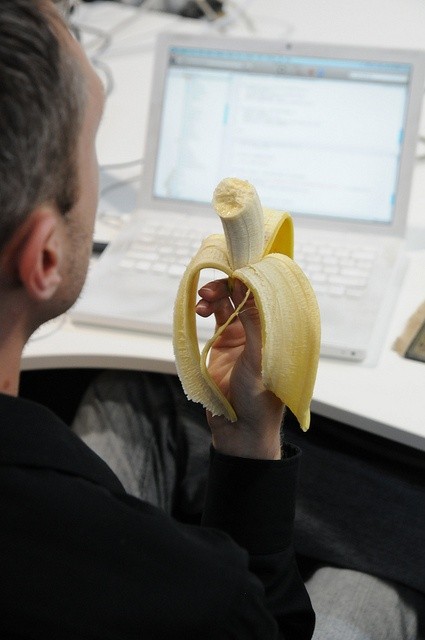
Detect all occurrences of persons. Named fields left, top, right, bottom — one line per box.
left=0, top=0, right=419, bottom=640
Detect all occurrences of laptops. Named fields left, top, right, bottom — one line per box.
left=71, top=29, right=425, bottom=362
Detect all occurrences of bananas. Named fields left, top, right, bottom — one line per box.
left=172, top=177, right=322, bottom=433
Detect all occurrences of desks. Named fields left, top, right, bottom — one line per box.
left=0, top=5, right=425, bottom=453
